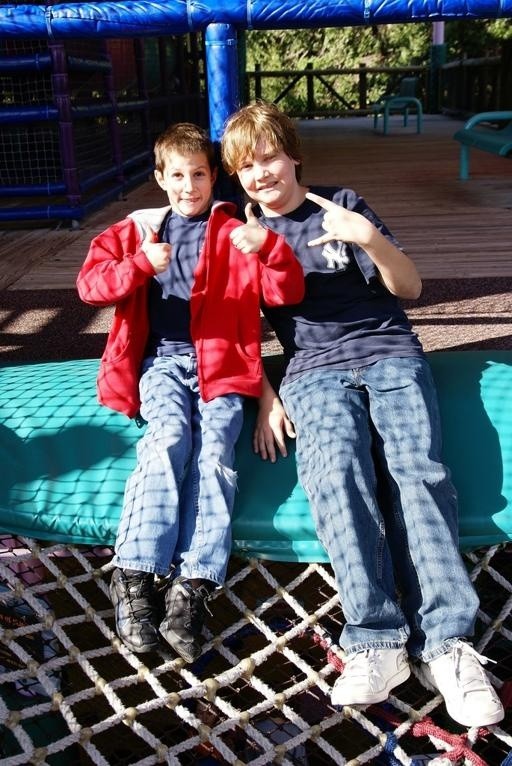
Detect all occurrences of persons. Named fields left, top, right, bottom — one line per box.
left=74, top=121, right=304, bottom=663
left=222, top=97, right=504, bottom=726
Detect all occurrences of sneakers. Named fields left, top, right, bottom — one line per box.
left=108, top=567, right=208, bottom=664
left=331, top=640, right=505, bottom=726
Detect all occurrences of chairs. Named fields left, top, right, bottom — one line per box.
left=453, top=111, right=512, bottom=182
left=373, top=75, right=424, bottom=134
